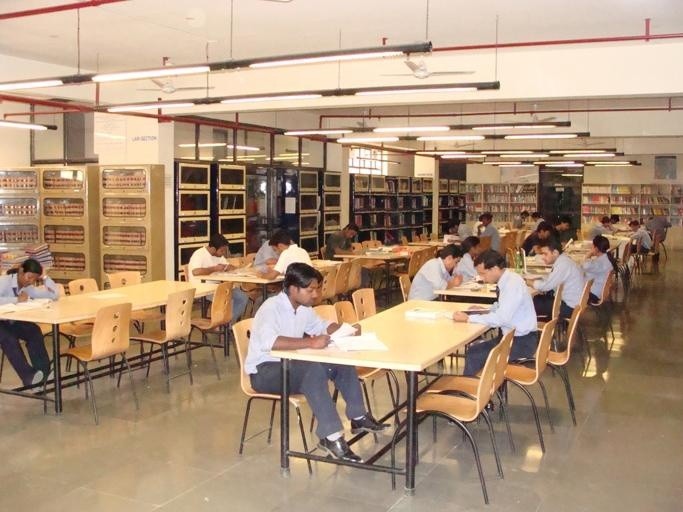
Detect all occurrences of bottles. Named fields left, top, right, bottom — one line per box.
left=513, top=250, right=521, bottom=274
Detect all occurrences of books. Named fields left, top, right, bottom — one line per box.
left=582, top=182, right=683, bottom=228
left=1, top=243, right=52, bottom=268
left=354, top=175, right=537, bottom=251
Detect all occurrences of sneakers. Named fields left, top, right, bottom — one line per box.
left=45, top=369, right=56, bottom=383
left=22, top=370, right=44, bottom=388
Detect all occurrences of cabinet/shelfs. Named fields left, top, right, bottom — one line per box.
left=1, top=164, right=165, bottom=288
left=232, top=278, right=594, bottom=505
left=175, top=161, right=341, bottom=283
left=349, top=174, right=432, bottom=245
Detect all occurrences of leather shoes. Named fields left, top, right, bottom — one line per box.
left=316, top=433, right=364, bottom=464
left=350, top=412, right=392, bottom=435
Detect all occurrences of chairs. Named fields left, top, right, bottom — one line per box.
left=433, top=158, right=682, bottom=227
left=183, top=229, right=667, bottom=356
left=1, top=270, right=233, bottom=425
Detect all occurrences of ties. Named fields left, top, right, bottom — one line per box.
left=496, top=286, right=503, bottom=338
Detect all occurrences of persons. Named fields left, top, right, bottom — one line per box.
left=406, top=242, right=463, bottom=300
left=513, top=211, right=673, bottom=266
left=187, top=233, right=249, bottom=331
left=579, top=234, right=619, bottom=303
left=448, top=248, right=538, bottom=427
left=0, top=259, right=59, bottom=394
left=252, top=238, right=295, bottom=294
left=524, top=237, right=587, bottom=323
left=323, top=224, right=359, bottom=303
left=250, top=231, right=313, bottom=319
left=242, top=262, right=392, bottom=463
left=450, top=235, right=479, bottom=285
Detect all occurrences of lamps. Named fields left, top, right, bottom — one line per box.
left=0, top=43, right=432, bottom=91
left=0, top=121, right=58, bottom=130
left=92, top=81, right=499, bottom=113
left=328, top=133, right=590, bottom=143
left=416, top=149, right=640, bottom=168
left=275, top=121, right=571, bottom=135
left=179, top=143, right=262, bottom=152
